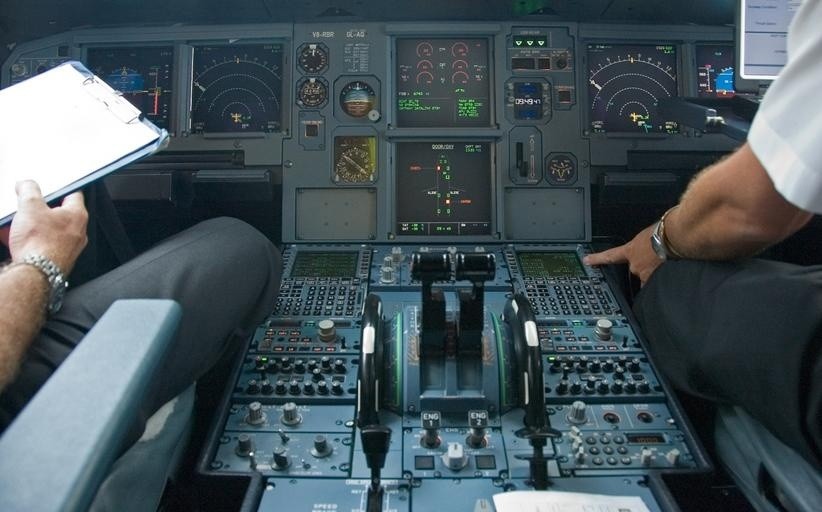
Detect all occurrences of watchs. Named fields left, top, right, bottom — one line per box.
left=651, top=206, right=679, bottom=263
left=1, top=253, right=68, bottom=317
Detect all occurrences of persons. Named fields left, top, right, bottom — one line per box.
left=581, top=0, right=821, bottom=478
left=0, top=179, right=284, bottom=449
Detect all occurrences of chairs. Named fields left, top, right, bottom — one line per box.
left=713, top=401, right=822, bottom=512
left=1, top=297, right=198, bottom=511
